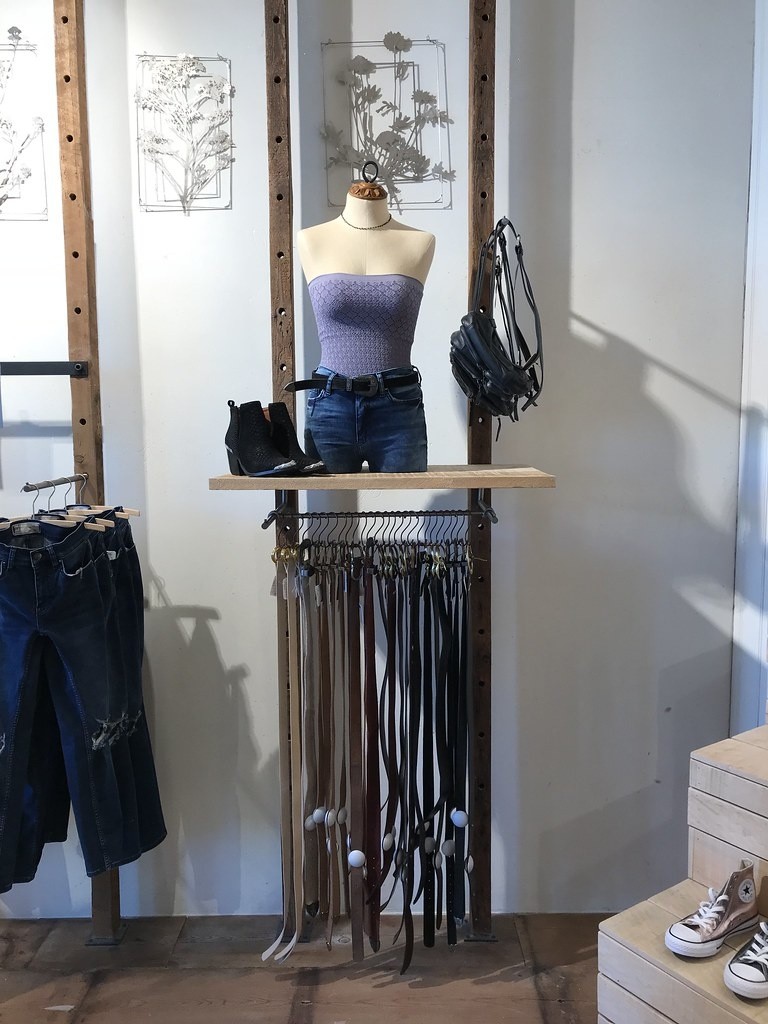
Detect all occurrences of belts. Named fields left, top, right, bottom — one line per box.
left=262, top=541, right=466, bottom=974
left=284, top=368, right=419, bottom=396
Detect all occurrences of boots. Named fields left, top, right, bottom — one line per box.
left=262, top=402, right=325, bottom=472
left=225, top=399, right=296, bottom=476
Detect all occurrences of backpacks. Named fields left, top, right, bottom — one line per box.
left=449, top=217, right=544, bottom=443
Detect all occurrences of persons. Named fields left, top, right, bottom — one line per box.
left=295, top=182, right=437, bottom=474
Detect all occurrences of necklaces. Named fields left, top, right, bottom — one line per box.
left=340, top=212, right=391, bottom=230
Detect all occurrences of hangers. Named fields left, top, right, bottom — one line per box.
left=268, top=505, right=487, bottom=582
left=0, top=473, right=140, bottom=533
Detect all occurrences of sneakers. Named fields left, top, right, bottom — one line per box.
left=665, top=858, right=759, bottom=958
left=723, top=921, right=768, bottom=999
